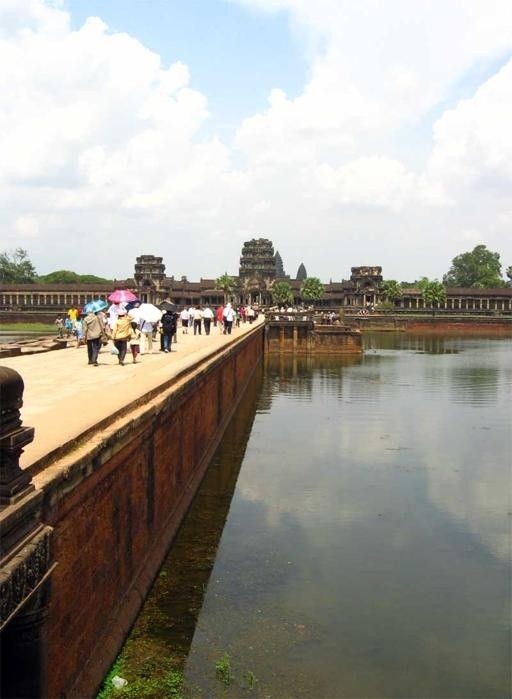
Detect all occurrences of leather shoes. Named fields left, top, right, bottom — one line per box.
left=88, top=360, right=99, bottom=366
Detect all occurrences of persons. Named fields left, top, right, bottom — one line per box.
left=51, top=300, right=258, bottom=364
left=268, top=304, right=336, bottom=326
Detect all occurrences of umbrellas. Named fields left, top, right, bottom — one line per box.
left=107, top=289, right=138, bottom=303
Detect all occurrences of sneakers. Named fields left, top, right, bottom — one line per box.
left=161, top=347, right=171, bottom=352
left=119, top=360, right=125, bottom=366
